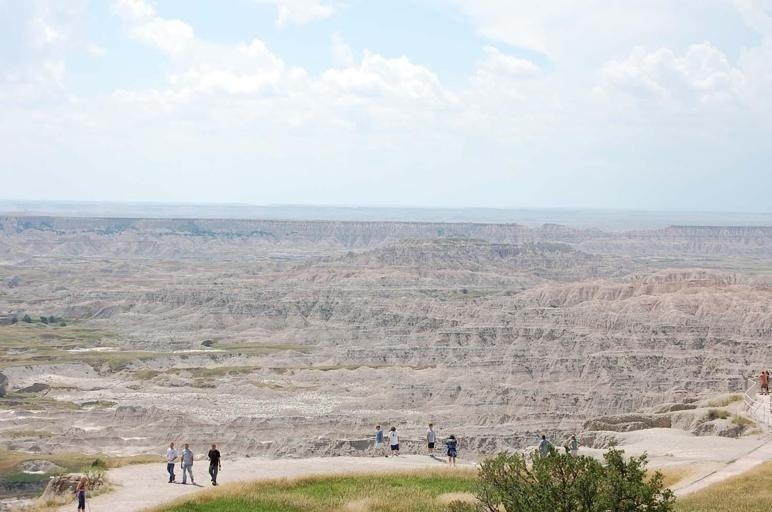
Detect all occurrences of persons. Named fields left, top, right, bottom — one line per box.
left=427, top=423, right=435, bottom=455
left=446, top=434, right=456, bottom=464
left=766, top=370, right=769, bottom=391
left=568, top=435, right=582, bottom=455
left=389, top=426, right=400, bottom=455
left=759, top=371, right=767, bottom=395
left=372, top=425, right=388, bottom=457
left=76, top=476, right=88, bottom=511
left=181, top=443, right=196, bottom=483
left=208, top=445, right=221, bottom=486
left=539, top=434, right=554, bottom=456
left=165, top=442, right=177, bottom=483
